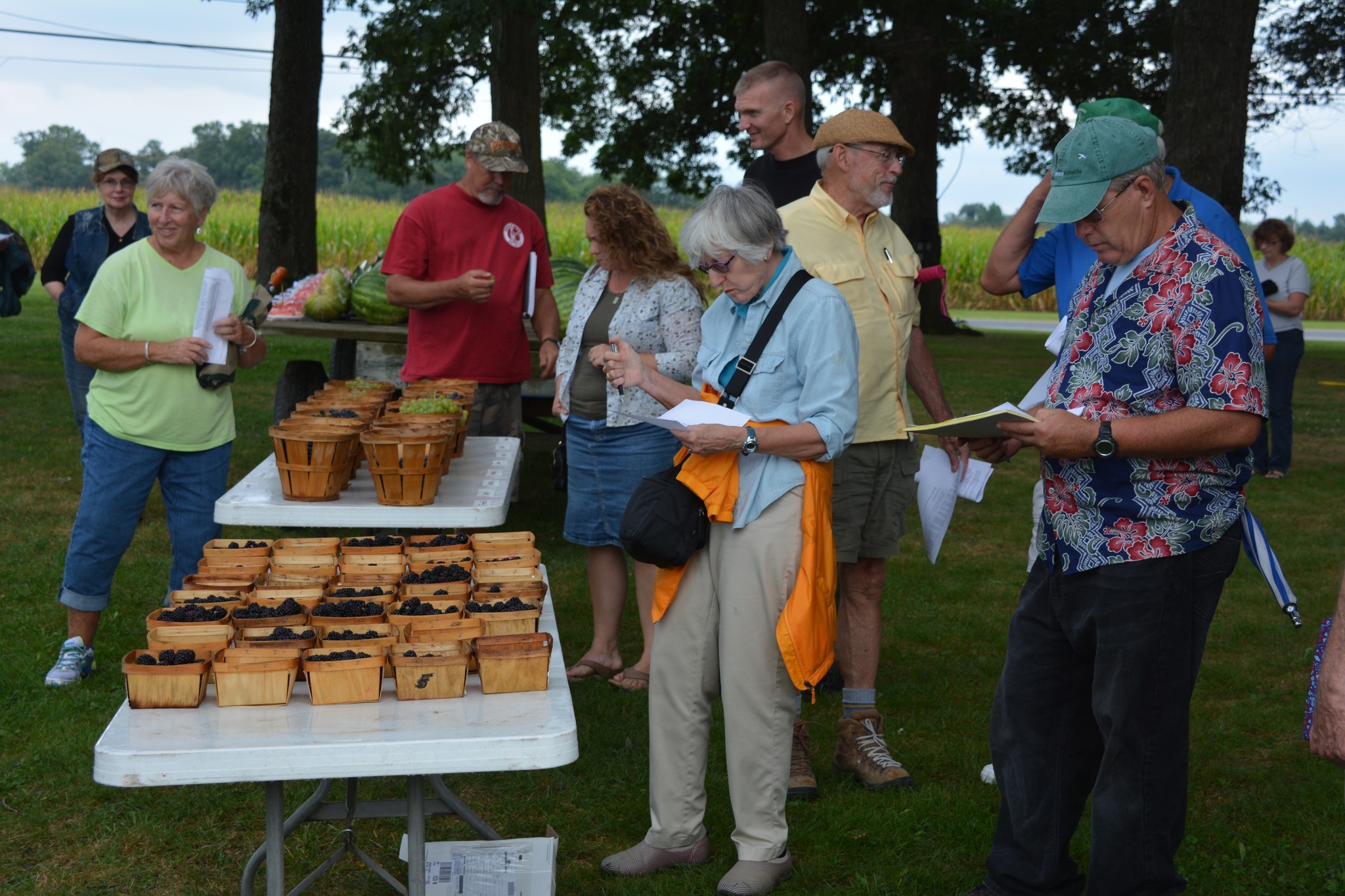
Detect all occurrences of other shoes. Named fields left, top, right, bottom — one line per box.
left=717, top=845, right=792, bottom=896
left=836, top=709, right=911, bottom=787
left=787, top=718, right=825, bottom=792
left=600, top=833, right=709, bottom=876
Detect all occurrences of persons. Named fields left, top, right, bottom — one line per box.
left=380, top=120, right=561, bottom=443
left=0, top=149, right=265, bottom=687
left=550, top=62, right=1345, bottom=896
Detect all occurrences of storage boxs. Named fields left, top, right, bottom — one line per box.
left=398, top=824, right=559, bottom=896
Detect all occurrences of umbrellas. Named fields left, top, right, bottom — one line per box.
left=1236, top=503, right=1303, bottom=627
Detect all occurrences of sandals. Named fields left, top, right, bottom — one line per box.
left=1264, top=470, right=1285, bottom=478
left=1251, top=470, right=1263, bottom=475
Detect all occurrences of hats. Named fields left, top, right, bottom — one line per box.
left=1033, top=115, right=1158, bottom=224
left=94, top=148, right=139, bottom=172
left=813, top=108, right=915, bottom=158
left=466, top=120, right=529, bottom=173
left=1074, top=98, right=1164, bottom=136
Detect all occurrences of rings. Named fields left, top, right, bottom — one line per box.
left=606, top=373, right=613, bottom=381
left=591, top=355, right=595, bottom=360
left=688, top=449, right=691, bottom=454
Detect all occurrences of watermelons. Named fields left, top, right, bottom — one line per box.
left=548, top=257, right=588, bottom=337
left=301, top=259, right=410, bottom=325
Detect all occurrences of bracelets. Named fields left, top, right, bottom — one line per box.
left=145, top=341, right=152, bottom=365
left=240, top=324, right=257, bottom=352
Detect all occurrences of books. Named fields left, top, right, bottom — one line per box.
left=523, top=251, right=538, bottom=319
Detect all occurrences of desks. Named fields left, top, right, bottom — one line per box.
left=212, top=437, right=521, bottom=535
left=257, top=320, right=543, bottom=381
left=92, top=564, right=579, bottom=896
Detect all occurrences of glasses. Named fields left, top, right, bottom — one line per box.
left=697, top=251, right=738, bottom=275
left=1259, top=239, right=1280, bottom=246
left=1083, top=176, right=1139, bottom=223
left=846, top=145, right=905, bottom=167
left=102, top=177, right=132, bottom=186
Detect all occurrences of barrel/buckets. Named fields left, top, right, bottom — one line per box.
left=267, top=380, right=478, bottom=507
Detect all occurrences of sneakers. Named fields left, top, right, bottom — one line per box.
left=44, top=636, right=97, bottom=687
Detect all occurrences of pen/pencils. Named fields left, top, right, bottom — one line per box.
left=884, top=248, right=893, bottom=264
left=964, top=412, right=1010, bottom=463
left=610, top=342, right=625, bottom=404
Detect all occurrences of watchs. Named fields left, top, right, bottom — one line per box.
left=1093, top=421, right=1119, bottom=461
left=741, top=425, right=759, bottom=456
left=542, top=338, right=561, bottom=349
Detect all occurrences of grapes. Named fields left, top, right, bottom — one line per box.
left=400, top=394, right=469, bottom=426
left=336, top=376, right=382, bottom=401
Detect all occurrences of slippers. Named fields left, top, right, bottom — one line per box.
left=608, top=667, right=650, bottom=693
left=566, top=660, right=624, bottom=682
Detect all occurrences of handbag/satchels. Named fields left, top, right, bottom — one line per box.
left=621, top=465, right=710, bottom=568
